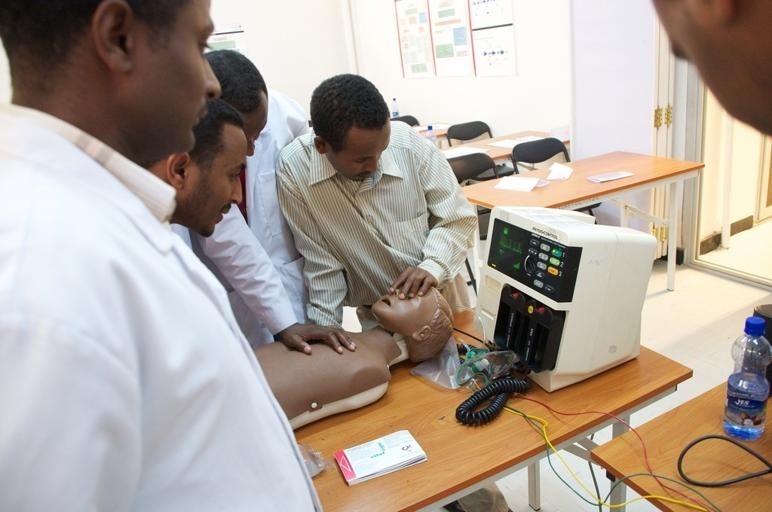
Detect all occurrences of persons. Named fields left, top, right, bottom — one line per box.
left=653, top=0, right=772, bottom=136
left=274, top=74, right=511, bottom=512
left=248, top=285, right=455, bottom=430
left=0, top=0, right=323, bottom=512
left=164, top=97, right=248, bottom=237
left=201, top=50, right=311, bottom=348
left=196, top=201, right=357, bottom=355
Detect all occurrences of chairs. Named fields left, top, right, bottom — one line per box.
left=391, top=114, right=602, bottom=227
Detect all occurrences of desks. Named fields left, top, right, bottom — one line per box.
left=291, top=306, right=694, bottom=511
left=461, top=151, right=705, bottom=291
left=589, top=382, right=771, bottom=512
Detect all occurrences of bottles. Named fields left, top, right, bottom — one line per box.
left=391, top=98, right=400, bottom=118
left=723, top=316, right=772, bottom=442
left=426, top=125, right=437, bottom=143
